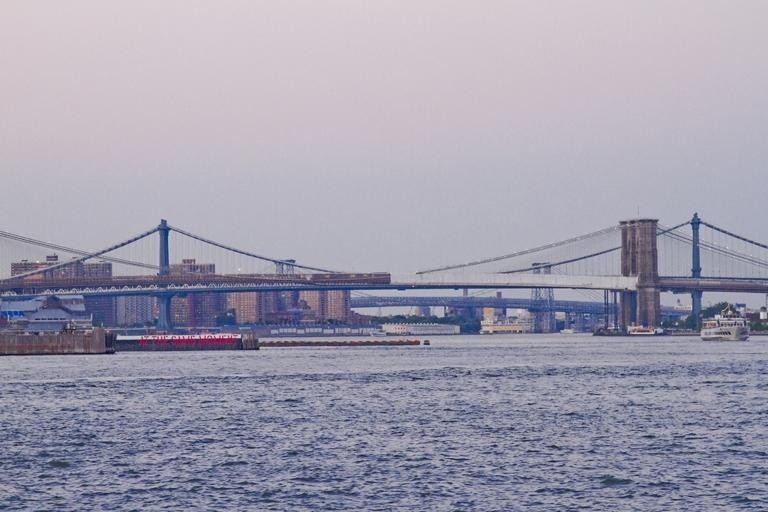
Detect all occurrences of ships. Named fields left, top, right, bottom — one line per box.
left=476, top=318, right=527, bottom=335
left=383, top=321, right=457, bottom=337
left=700, top=301, right=750, bottom=342
